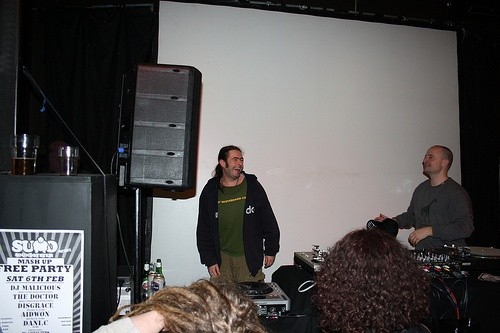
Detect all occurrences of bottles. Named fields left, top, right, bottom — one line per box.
left=155, top=258, right=166, bottom=289
left=139, top=261, right=154, bottom=303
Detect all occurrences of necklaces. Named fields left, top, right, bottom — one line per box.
left=235, top=177, right=239, bottom=187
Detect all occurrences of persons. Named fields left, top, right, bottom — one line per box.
left=374, top=145, right=475, bottom=251
left=310, top=229, right=432, bottom=333
left=196, top=145, right=281, bottom=283
left=91, top=273, right=273, bottom=333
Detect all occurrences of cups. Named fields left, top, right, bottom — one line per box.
left=9, top=133, right=40, bottom=175
left=57, top=145, right=80, bottom=176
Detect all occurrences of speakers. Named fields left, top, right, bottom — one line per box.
left=129, top=62, right=202, bottom=190
